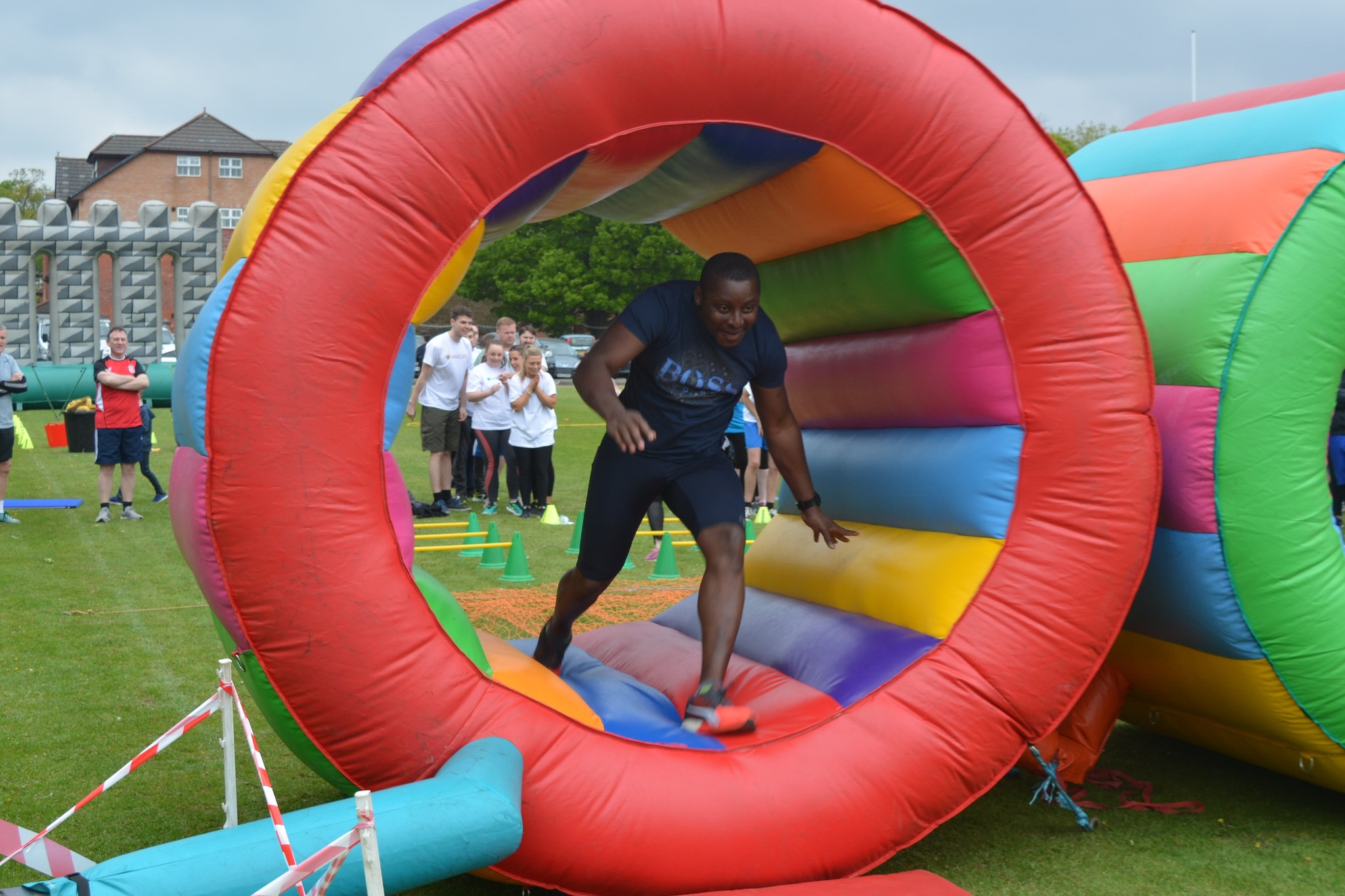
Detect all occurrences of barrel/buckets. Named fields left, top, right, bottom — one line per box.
left=44, top=422, right=71, bottom=447
left=62, top=410, right=96, bottom=453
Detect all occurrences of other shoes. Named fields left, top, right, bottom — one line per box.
left=769, top=508, right=777, bottom=518
left=751, top=502, right=761, bottom=516
left=744, top=507, right=751, bottom=518
left=758, top=500, right=767, bottom=507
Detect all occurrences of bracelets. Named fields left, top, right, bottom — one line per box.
left=525, top=388, right=532, bottom=396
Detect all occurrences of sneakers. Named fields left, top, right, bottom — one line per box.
left=645, top=548, right=659, bottom=561
left=432, top=500, right=450, bottom=514
left=467, top=491, right=475, bottom=497
left=95, top=507, right=111, bottom=523
left=517, top=496, right=524, bottom=510
left=457, top=494, right=469, bottom=505
left=506, top=501, right=523, bottom=516
left=446, top=498, right=472, bottom=511
left=521, top=505, right=531, bottom=519
left=121, top=506, right=144, bottom=520
left=681, top=688, right=757, bottom=736
left=482, top=503, right=497, bottom=514
left=532, top=615, right=572, bottom=677
left=531, top=502, right=538, bottom=514
left=0, top=511, right=21, bottom=524
left=472, top=488, right=499, bottom=502
left=537, top=505, right=546, bottom=518
left=109, top=494, right=123, bottom=505
left=152, top=492, right=168, bottom=503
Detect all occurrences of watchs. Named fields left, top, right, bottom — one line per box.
left=794, top=492, right=821, bottom=511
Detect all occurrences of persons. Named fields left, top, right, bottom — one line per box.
left=93, top=327, right=149, bottom=523
left=109, top=388, right=168, bottom=505
left=0, top=322, right=28, bottom=524
left=646, top=496, right=668, bottom=561
left=723, top=379, right=780, bottom=519
left=414, top=334, right=430, bottom=373
left=459, top=315, right=557, bottom=520
left=405, top=305, right=473, bottom=514
left=534, top=251, right=858, bottom=734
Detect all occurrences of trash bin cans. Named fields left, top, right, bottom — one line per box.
left=62, top=411, right=96, bottom=453
